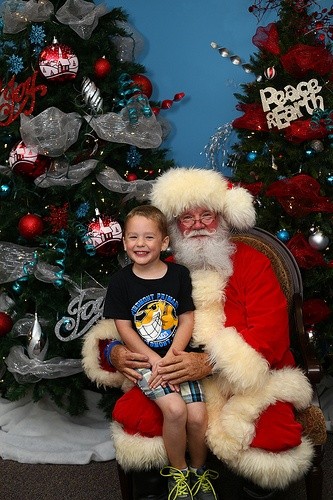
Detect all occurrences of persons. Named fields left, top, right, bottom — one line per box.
left=80, top=166, right=316, bottom=499
left=102, top=205, right=219, bottom=500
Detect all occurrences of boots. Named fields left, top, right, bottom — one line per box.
left=226, top=437, right=314, bottom=500
left=109, top=421, right=169, bottom=500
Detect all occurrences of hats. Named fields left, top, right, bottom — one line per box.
left=150, top=166, right=255, bottom=230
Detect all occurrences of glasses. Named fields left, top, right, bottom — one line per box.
left=176, top=212, right=219, bottom=227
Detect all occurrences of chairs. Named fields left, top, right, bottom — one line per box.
left=116, top=226, right=323, bottom=499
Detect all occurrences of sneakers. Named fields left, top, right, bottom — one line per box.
left=160, top=466, right=193, bottom=500
left=189, top=464, right=219, bottom=500
